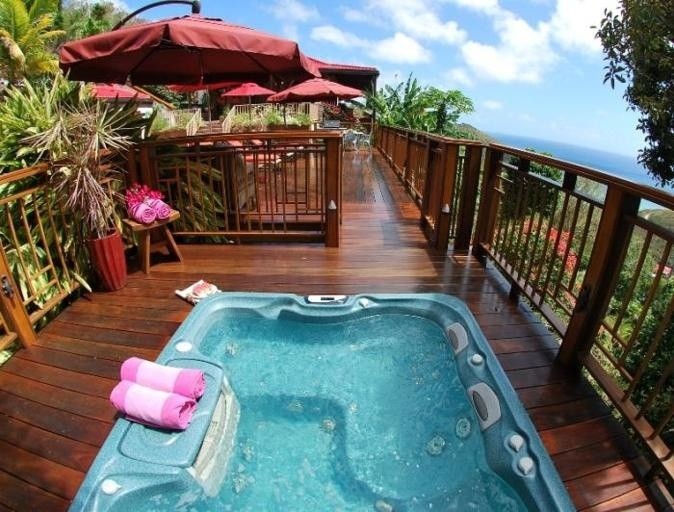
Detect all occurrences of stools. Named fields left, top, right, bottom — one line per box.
left=122, top=209, right=183, bottom=273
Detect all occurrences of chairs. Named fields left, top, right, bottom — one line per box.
left=343, top=125, right=373, bottom=151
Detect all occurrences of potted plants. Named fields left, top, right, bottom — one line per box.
left=17, top=98, right=139, bottom=293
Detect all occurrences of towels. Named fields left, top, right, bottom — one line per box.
left=125, top=181, right=171, bottom=223
left=110, top=380, right=197, bottom=430
left=120, top=356, right=206, bottom=399
left=174, top=278, right=224, bottom=307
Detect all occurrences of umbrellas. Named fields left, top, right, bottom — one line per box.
left=165, top=81, right=241, bottom=134
left=59, top=13, right=322, bottom=133
left=91, top=82, right=150, bottom=99
left=266, top=76, right=363, bottom=121
left=222, top=82, right=277, bottom=133
